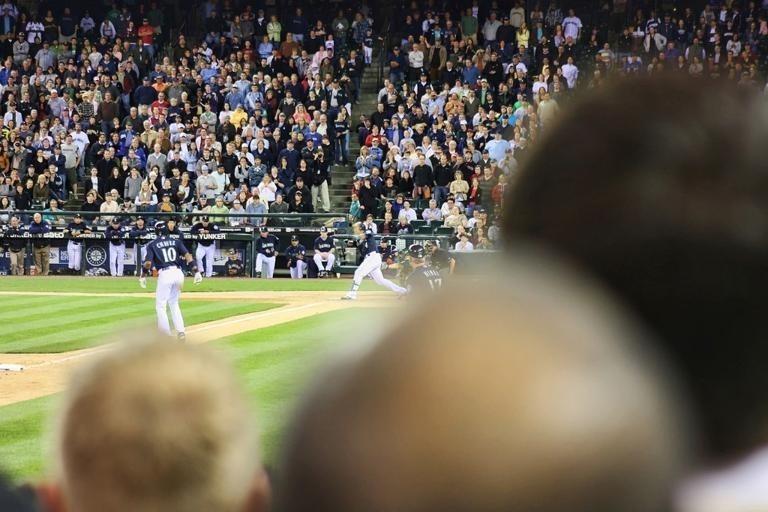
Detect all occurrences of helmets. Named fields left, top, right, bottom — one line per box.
left=155, top=221, right=167, bottom=233
left=74, top=214, right=208, bottom=224
left=260, top=226, right=327, bottom=240
left=408, top=244, right=425, bottom=258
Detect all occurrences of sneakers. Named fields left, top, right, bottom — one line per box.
left=178, top=332, right=185, bottom=342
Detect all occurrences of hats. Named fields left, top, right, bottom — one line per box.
left=0, top=0, right=579, bottom=211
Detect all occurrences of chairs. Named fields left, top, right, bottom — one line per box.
left=1, top=1, right=768, bottom=277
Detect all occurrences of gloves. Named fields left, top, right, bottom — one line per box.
left=139, top=276, right=146, bottom=288
left=193, top=272, right=202, bottom=286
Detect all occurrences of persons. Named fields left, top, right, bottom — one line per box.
left=0, top=1, right=768, bottom=511
